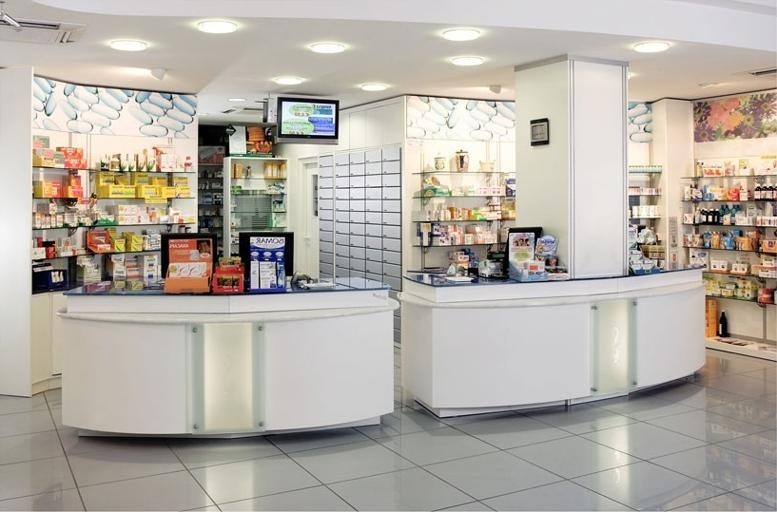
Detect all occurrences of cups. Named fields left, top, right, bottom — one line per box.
left=434, top=157, right=446, bottom=170
left=479, top=159, right=496, bottom=172
left=456, top=150, right=469, bottom=171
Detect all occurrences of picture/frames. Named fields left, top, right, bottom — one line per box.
left=529, top=118, right=550, bottom=145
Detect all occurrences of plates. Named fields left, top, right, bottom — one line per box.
left=445, top=276, right=475, bottom=284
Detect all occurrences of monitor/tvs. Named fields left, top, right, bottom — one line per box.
left=276, top=95, right=340, bottom=144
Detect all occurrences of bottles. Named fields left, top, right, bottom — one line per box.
left=695, top=201, right=741, bottom=225
left=718, top=309, right=727, bottom=337
left=185, top=156, right=192, bottom=171
left=204, top=168, right=208, bottom=178
left=753, top=181, right=777, bottom=199
left=703, top=230, right=725, bottom=248
left=247, top=165, right=253, bottom=177
left=109, top=154, right=119, bottom=171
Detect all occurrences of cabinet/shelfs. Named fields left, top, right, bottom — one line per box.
left=200, top=153, right=288, bottom=259
left=624, top=171, right=777, bottom=304
left=31, top=165, right=196, bottom=263
left=410, top=171, right=516, bottom=247
